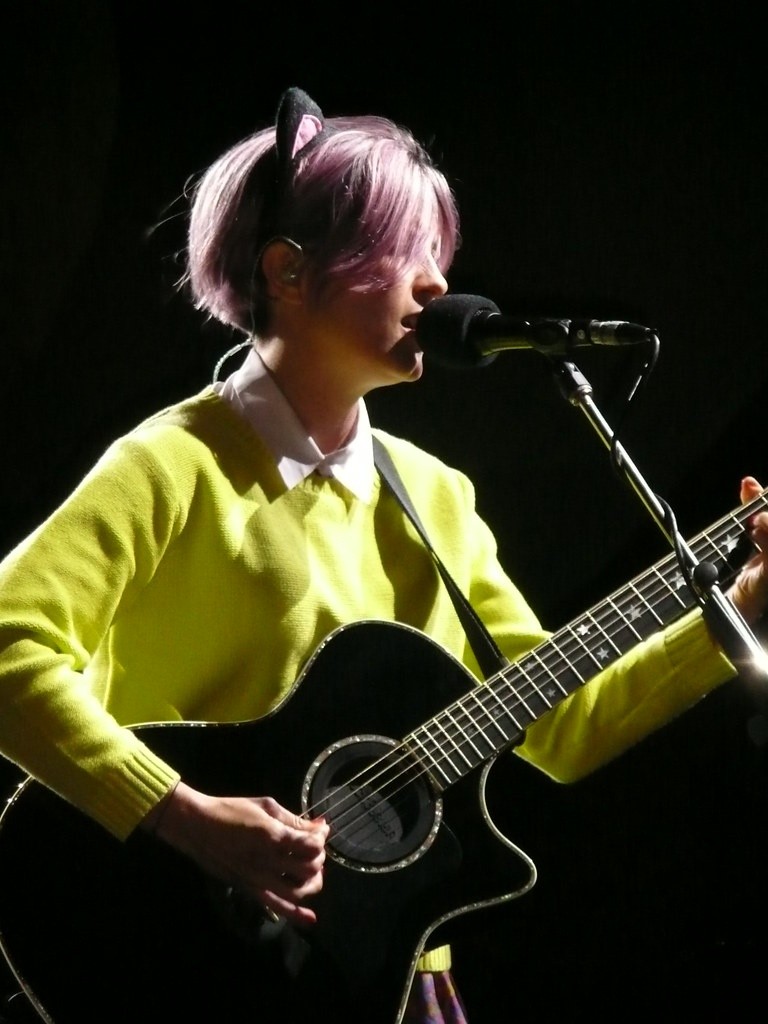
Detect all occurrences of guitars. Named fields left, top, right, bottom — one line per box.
left=0, top=489, right=768, bottom=1023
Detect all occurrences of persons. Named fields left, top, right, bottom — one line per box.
left=0, top=86, right=768, bottom=1023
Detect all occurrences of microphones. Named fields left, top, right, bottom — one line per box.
left=417, top=294, right=655, bottom=368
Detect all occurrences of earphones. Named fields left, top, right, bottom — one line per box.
left=278, top=261, right=306, bottom=286
